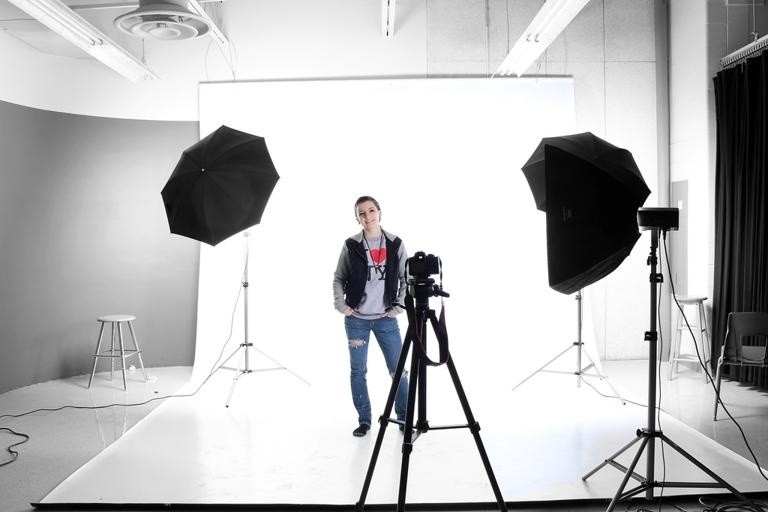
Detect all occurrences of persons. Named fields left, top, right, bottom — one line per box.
left=333, top=196, right=414, bottom=437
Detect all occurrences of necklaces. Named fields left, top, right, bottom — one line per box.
left=364, top=234, right=382, bottom=274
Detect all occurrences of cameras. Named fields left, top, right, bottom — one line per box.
left=409, top=251, right=439, bottom=275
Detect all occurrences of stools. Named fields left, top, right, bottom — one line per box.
left=670, top=296, right=712, bottom=384
left=85, top=313, right=147, bottom=390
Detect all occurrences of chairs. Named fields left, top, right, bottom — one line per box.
left=713, top=304, right=768, bottom=420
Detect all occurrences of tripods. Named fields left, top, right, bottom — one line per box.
left=355, top=279, right=508, bottom=512
left=513, top=289, right=628, bottom=407
left=581, top=229, right=766, bottom=512
left=202, top=233, right=313, bottom=408
